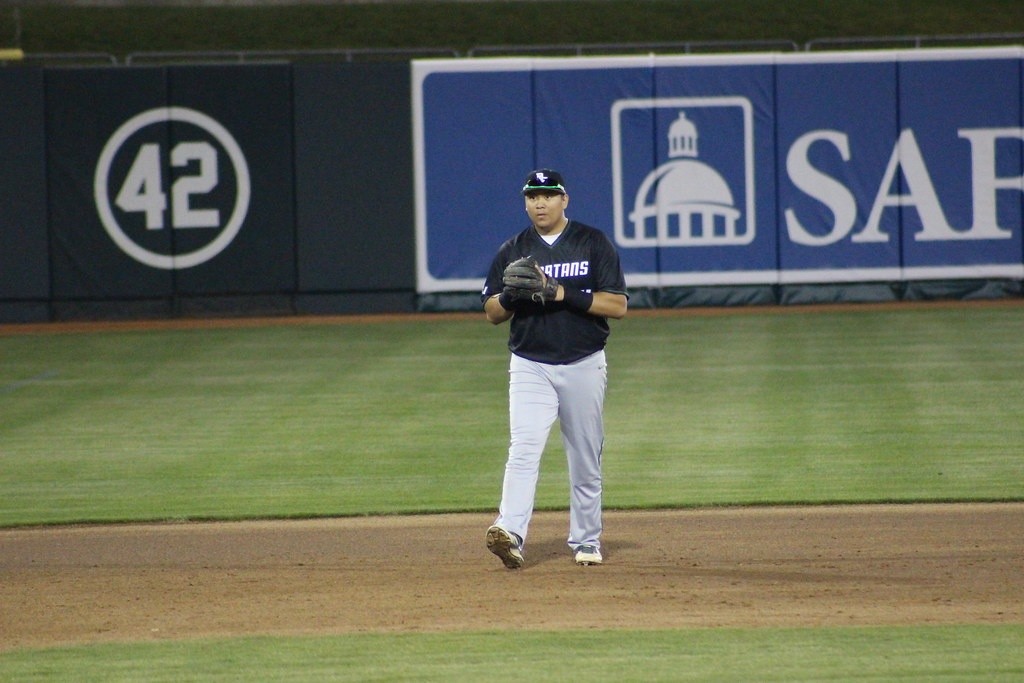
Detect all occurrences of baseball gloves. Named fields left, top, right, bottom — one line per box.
left=498, top=255, right=562, bottom=314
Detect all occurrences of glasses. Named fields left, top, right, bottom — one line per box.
left=527, top=178, right=558, bottom=187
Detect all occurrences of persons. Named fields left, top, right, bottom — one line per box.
left=480, top=169, right=631, bottom=570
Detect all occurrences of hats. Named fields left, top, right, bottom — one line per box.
left=521, top=168, right=566, bottom=194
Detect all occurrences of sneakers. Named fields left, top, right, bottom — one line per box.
left=574, top=545, right=602, bottom=566
left=485, top=526, right=524, bottom=569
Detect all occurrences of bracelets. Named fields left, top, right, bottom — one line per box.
left=561, top=287, right=594, bottom=314
